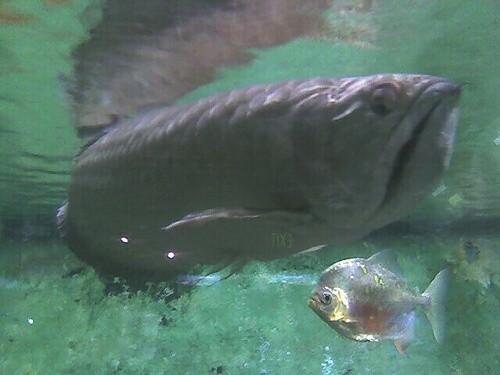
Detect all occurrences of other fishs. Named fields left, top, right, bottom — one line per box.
left=307, top=248, right=452, bottom=360
left=53, top=70, right=460, bottom=285
left=71, top=1, right=370, bottom=137
